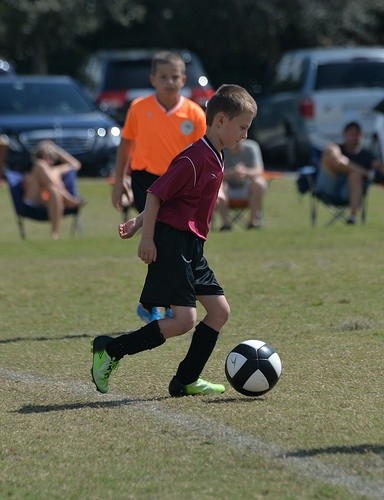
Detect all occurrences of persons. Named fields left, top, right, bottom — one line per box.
left=91, top=84, right=258, bottom=396
left=112, top=51, right=207, bottom=214
left=311, top=121, right=384, bottom=226
left=216, top=139, right=267, bottom=232
left=24, top=139, right=88, bottom=240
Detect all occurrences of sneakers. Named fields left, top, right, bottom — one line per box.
left=169, top=375, right=226, bottom=397
left=90, top=335, right=119, bottom=394
left=137, top=304, right=173, bottom=324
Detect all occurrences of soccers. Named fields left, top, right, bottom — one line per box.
left=225, top=339, right=283, bottom=396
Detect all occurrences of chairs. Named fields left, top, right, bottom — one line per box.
left=295, top=142, right=375, bottom=227
left=3, top=167, right=86, bottom=241
left=226, top=170, right=284, bottom=231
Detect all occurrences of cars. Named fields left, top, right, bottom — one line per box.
left=0, top=74, right=123, bottom=176
left=250, top=45, right=384, bottom=170
left=73, top=47, right=216, bottom=133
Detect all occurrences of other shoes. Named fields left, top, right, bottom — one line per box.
left=347, top=220, right=354, bottom=224
left=248, top=223, right=261, bottom=228
left=220, top=225, right=230, bottom=231
left=77, top=200, right=87, bottom=208
left=375, top=171, right=384, bottom=183
